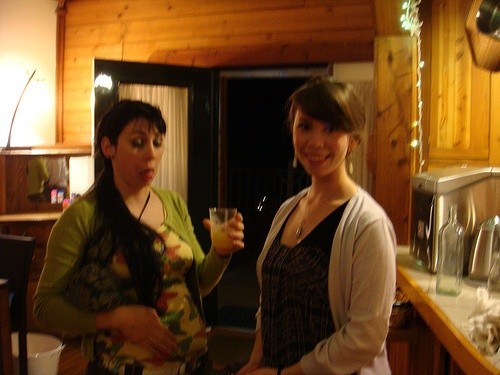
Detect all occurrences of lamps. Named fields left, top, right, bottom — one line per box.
left=2, top=58, right=45, bottom=150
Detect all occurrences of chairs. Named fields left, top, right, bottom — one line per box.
left=0, top=234, right=35, bottom=375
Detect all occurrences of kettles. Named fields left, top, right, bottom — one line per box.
left=468, top=215, right=500, bottom=282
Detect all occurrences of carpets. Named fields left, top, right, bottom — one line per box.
left=209, top=333, right=253, bottom=365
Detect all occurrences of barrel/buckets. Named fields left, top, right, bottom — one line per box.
left=10, top=332, right=63, bottom=375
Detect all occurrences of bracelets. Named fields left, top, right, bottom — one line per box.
left=277, top=368, right=282, bottom=375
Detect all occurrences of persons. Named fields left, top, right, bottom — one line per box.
left=34, top=100, right=245, bottom=375
left=236, top=80, right=397, bottom=375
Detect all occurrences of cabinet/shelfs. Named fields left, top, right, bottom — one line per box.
left=0, top=145, right=91, bottom=246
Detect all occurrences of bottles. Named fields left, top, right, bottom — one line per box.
left=435, top=203, right=465, bottom=297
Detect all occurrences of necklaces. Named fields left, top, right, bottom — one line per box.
left=138, top=191, right=150, bottom=221
left=295, top=224, right=302, bottom=239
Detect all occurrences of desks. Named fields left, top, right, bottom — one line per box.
left=396, top=245, right=500, bottom=375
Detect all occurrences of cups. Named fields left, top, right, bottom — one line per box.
left=209, top=208, right=237, bottom=256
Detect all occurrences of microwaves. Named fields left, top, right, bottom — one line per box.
left=409, top=166, right=500, bottom=274
